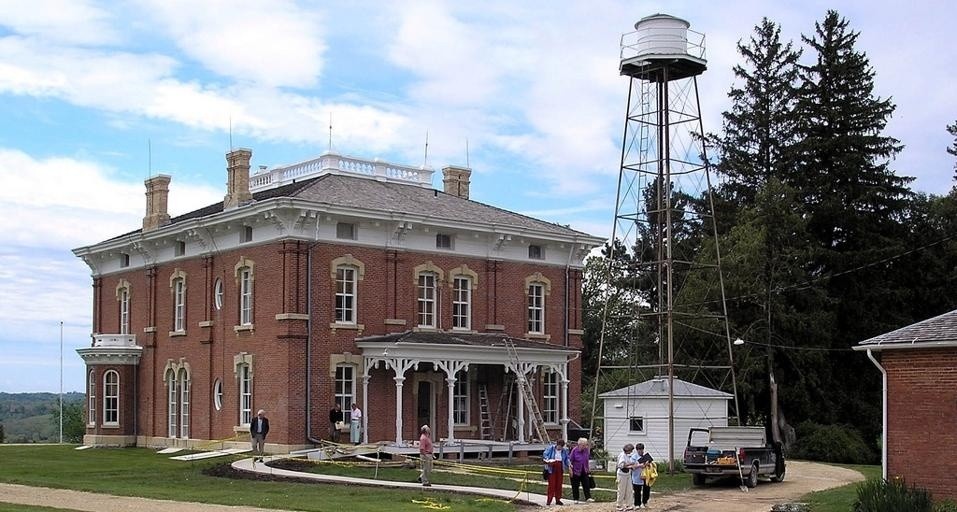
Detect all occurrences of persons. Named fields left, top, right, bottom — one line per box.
left=616, top=443, right=638, bottom=511
left=543, top=439, right=574, bottom=506
left=329, top=403, right=343, bottom=442
left=631, top=443, right=655, bottom=511
left=350, top=403, right=362, bottom=445
left=250, top=408, right=269, bottom=462
left=569, top=437, right=596, bottom=502
left=418, top=424, right=435, bottom=487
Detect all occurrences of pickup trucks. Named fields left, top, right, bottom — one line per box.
left=678, top=417, right=790, bottom=494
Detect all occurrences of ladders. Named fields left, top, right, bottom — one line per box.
left=503, top=338, right=552, bottom=445
left=478, top=384, right=494, bottom=439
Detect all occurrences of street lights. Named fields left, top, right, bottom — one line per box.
left=55, top=319, right=66, bottom=446
left=733, top=314, right=775, bottom=445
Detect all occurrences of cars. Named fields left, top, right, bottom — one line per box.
left=556, top=416, right=595, bottom=445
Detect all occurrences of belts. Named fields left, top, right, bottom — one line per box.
left=256, top=431, right=262, bottom=434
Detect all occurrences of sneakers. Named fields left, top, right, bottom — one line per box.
left=254, top=457, right=264, bottom=463
left=587, top=498, right=595, bottom=503
left=574, top=500, right=579, bottom=504
left=617, top=503, right=646, bottom=511
left=417, top=477, right=432, bottom=487
left=556, top=501, right=563, bottom=505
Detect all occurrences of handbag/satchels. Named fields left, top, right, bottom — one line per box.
left=541, top=467, right=551, bottom=481
left=587, top=473, right=597, bottom=489
